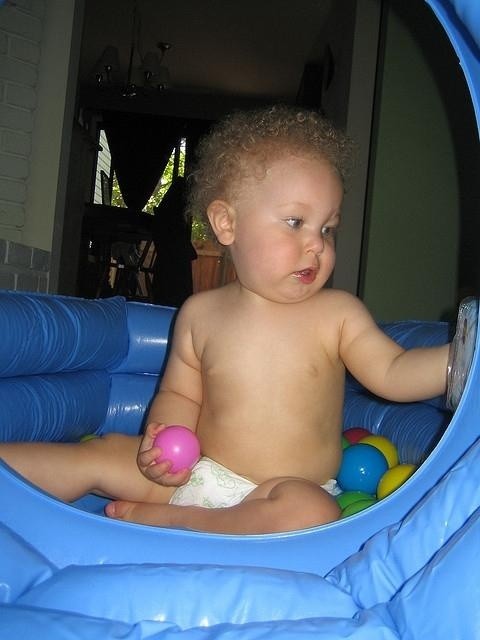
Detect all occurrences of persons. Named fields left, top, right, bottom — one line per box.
left=0, top=104, right=461, bottom=538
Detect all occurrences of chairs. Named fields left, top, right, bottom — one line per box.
left=98, top=166, right=186, bottom=300
